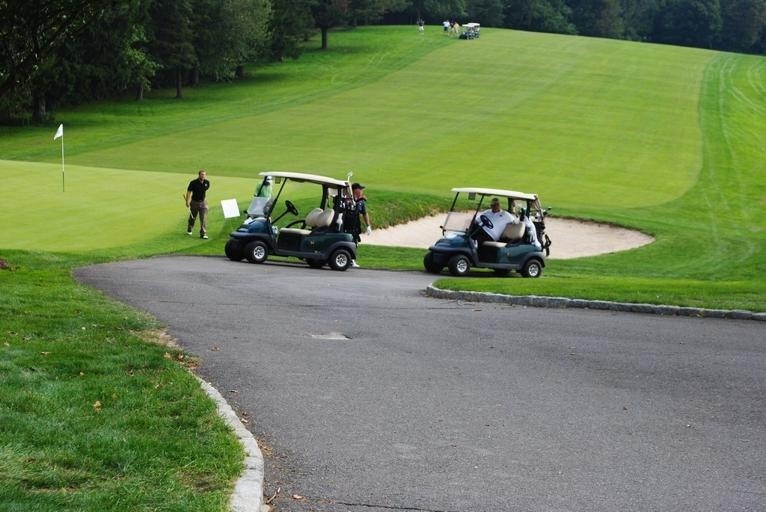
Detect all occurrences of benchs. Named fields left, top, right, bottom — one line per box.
left=278, top=207, right=335, bottom=252
left=479, top=222, right=525, bottom=262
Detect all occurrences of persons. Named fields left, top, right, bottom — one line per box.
left=343, top=181, right=372, bottom=268
left=417, top=17, right=460, bottom=36
left=460, top=197, right=516, bottom=243
left=254, top=174, right=272, bottom=198
left=184, top=168, right=211, bottom=240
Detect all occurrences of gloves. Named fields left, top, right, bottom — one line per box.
left=367, top=225, right=371, bottom=236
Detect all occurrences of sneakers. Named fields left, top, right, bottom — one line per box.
left=187, top=229, right=209, bottom=239
left=351, top=259, right=361, bottom=268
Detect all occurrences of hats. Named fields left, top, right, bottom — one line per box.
left=491, top=198, right=499, bottom=205
left=351, top=182, right=365, bottom=190
